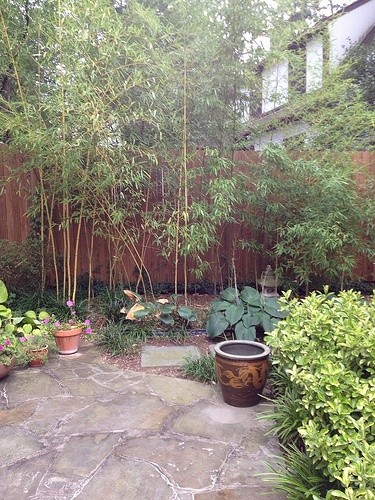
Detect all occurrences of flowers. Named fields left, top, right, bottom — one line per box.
left=43, top=301, right=93, bottom=335
left=0, top=333, right=57, bottom=367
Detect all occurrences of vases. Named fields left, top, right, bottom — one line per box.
left=0, top=354, right=15, bottom=378
left=215, top=340, right=270, bottom=408
left=23, top=344, right=49, bottom=367
left=53, top=325, right=82, bottom=354
